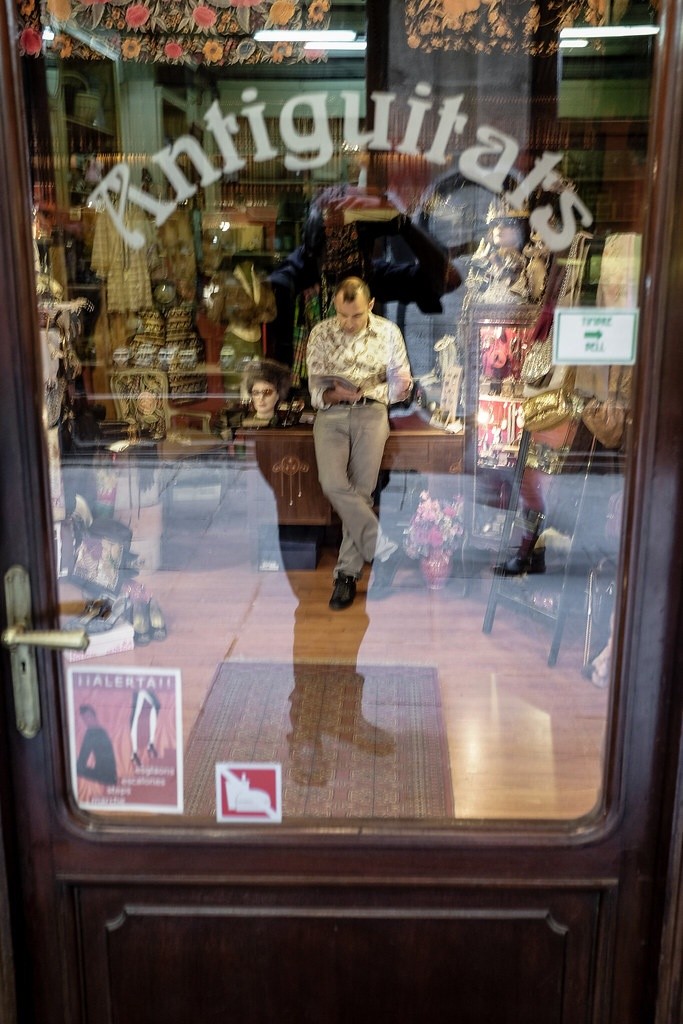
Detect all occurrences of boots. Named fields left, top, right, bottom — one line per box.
left=326, top=673, right=394, bottom=755
left=285, top=677, right=328, bottom=786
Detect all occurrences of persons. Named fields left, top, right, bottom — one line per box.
left=301, top=276, right=415, bottom=611
left=489, top=458, right=547, bottom=574
left=238, top=360, right=287, bottom=420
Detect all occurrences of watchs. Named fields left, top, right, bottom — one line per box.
left=405, top=379, right=414, bottom=398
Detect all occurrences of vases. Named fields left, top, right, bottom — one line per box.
left=419, top=546, right=453, bottom=590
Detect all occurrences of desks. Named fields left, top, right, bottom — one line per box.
left=235, top=408, right=465, bottom=573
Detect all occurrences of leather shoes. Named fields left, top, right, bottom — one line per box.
left=490, top=536, right=547, bottom=577
left=367, top=545, right=407, bottom=601
left=328, top=571, right=357, bottom=609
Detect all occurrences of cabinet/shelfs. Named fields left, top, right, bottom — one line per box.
left=460, top=299, right=545, bottom=598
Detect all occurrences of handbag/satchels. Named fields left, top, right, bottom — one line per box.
left=511, top=236, right=632, bottom=454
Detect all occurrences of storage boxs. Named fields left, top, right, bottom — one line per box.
left=260, top=528, right=319, bottom=570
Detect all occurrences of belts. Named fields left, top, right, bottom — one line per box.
left=339, top=396, right=376, bottom=406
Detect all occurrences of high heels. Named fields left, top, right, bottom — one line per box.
left=63, top=598, right=111, bottom=634
left=86, top=596, right=134, bottom=634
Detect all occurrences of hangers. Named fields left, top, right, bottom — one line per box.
left=309, top=176, right=408, bottom=216
left=93, top=178, right=149, bottom=218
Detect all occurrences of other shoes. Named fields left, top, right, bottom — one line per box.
left=147, top=599, right=168, bottom=642
left=131, top=604, right=150, bottom=646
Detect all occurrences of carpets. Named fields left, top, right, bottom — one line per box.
left=149, top=659, right=462, bottom=826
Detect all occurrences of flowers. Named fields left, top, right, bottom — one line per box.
left=397, top=485, right=470, bottom=560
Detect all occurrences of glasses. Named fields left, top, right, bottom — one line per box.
left=251, top=388, right=275, bottom=397
left=489, top=217, right=528, bottom=230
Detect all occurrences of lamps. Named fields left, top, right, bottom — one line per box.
left=40, top=24, right=56, bottom=41
left=252, top=30, right=370, bottom=52
left=559, top=25, right=661, bottom=48
